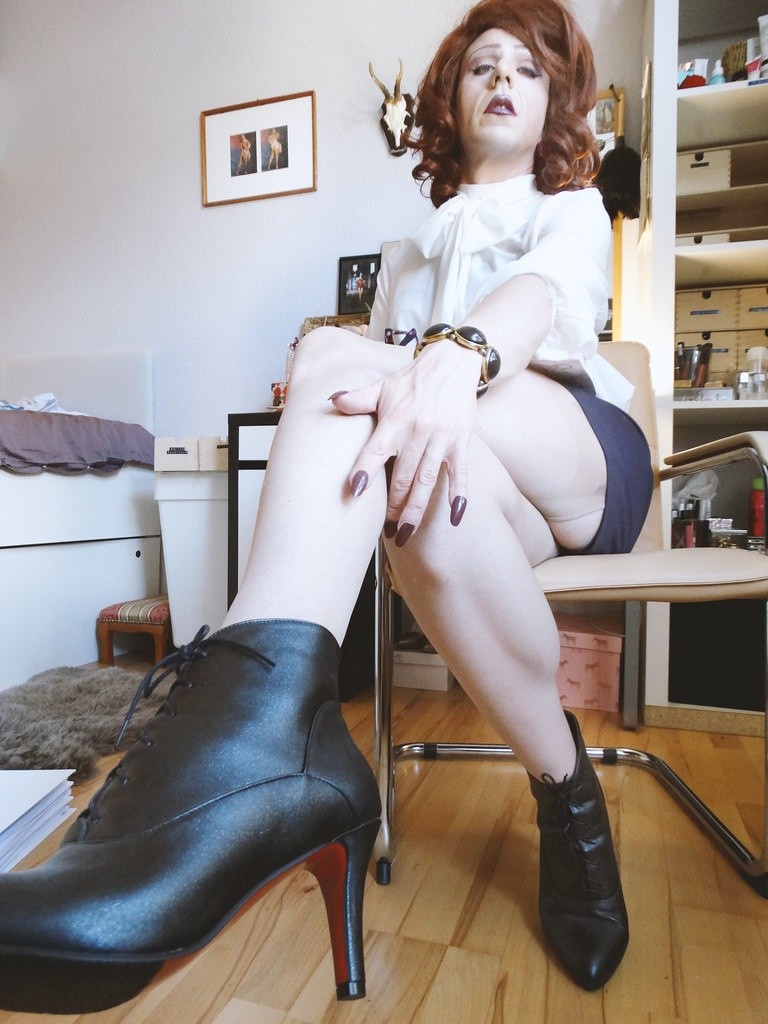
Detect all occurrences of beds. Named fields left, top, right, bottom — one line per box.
left=0, top=349, right=160, bottom=693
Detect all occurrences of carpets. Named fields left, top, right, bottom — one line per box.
left=0, top=666, right=174, bottom=785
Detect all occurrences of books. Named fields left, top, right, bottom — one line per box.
left=0, top=770, right=79, bottom=875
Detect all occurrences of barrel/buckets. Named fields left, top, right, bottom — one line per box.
left=675, top=344, right=711, bottom=397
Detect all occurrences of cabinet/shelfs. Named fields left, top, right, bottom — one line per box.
left=649, top=0, right=768, bottom=739
left=227, top=412, right=375, bottom=703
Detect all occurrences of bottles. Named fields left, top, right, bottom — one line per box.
left=710, top=60, right=725, bottom=85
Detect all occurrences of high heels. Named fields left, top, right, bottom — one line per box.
left=524, top=711, right=629, bottom=991
left=0, top=620, right=383, bottom=1014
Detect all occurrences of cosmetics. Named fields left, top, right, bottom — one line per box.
left=670, top=479, right=765, bottom=551
left=674, top=342, right=714, bottom=388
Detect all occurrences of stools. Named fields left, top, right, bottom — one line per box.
left=96, top=595, right=171, bottom=669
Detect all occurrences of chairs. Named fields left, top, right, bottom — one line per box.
left=374, top=340, right=768, bottom=898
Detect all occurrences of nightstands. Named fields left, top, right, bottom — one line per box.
left=153, top=470, right=229, bottom=648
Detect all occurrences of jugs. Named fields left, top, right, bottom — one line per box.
left=749, top=478, right=766, bottom=537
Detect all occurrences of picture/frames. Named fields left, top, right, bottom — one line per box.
left=201, top=90, right=317, bottom=207
left=338, top=254, right=382, bottom=315
left=588, top=87, right=626, bottom=342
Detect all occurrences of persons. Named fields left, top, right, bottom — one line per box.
left=0, top=0, right=650, bottom=1013
left=355, top=272, right=365, bottom=302
left=268, top=128, right=283, bottom=169
left=234, top=134, right=251, bottom=175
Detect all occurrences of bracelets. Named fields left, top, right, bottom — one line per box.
left=413, top=323, right=501, bottom=400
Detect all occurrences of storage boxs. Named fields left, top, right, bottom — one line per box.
left=390, top=650, right=457, bottom=691
left=675, top=233, right=730, bottom=247
left=674, top=287, right=768, bottom=387
left=676, top=149, right=732, bottom=192
left=552, top=611, right=624, bottom=712
